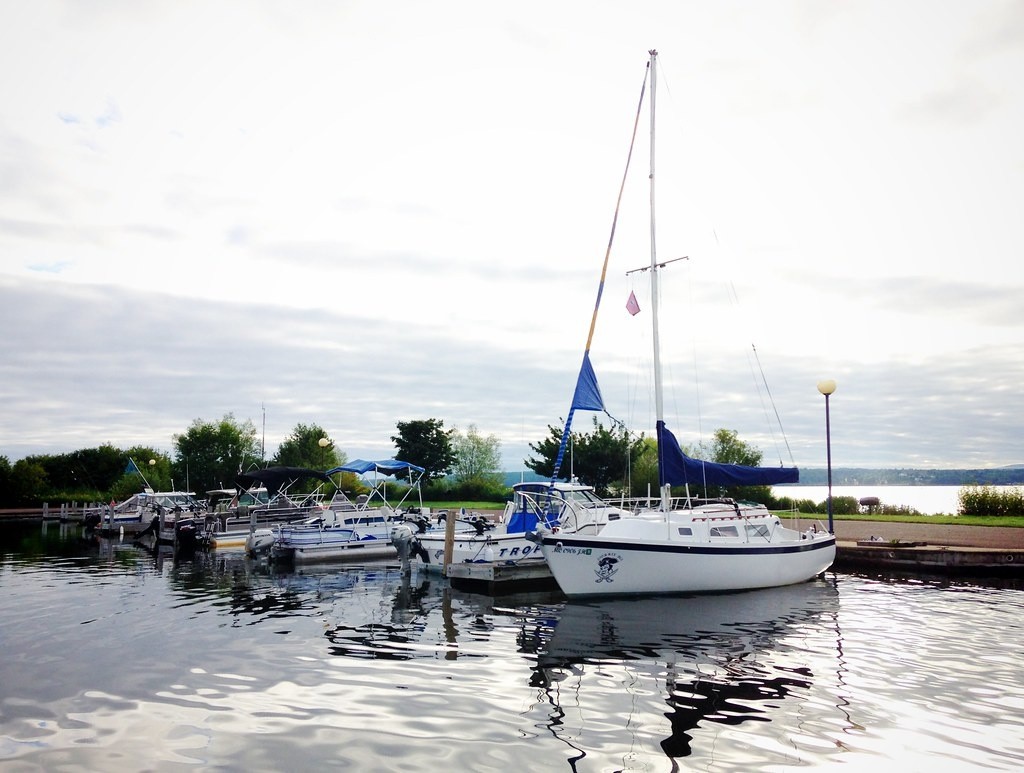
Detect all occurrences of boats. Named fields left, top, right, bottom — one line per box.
left=388, top=481, right=631, bottom=571
left=221, top=488, right=222, bottom=493
left=246, top=493, right=496, bottom=560
left=83, top=493, right=358, bottom=554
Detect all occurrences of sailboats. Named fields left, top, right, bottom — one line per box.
left=527, top=46, right=837, bottom=598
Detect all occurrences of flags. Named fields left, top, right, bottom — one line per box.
left=626, top=290, right=640, bottom=316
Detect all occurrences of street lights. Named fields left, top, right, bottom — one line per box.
left=150, top=460, right=156, bottom=493
left=318, top=437, right=329, bottom=493
left=816, top=377, right=837, bottom=537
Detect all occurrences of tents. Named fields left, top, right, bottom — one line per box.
left=325, top=459, right=425, bottom=512
left=227, top=466, right=331, bottom=509
